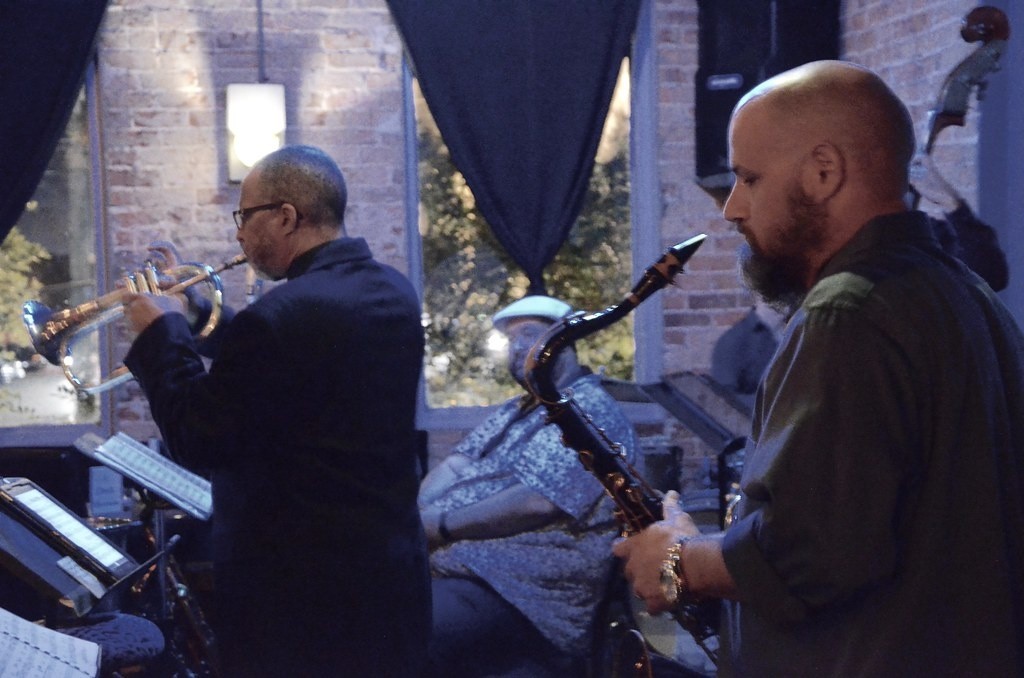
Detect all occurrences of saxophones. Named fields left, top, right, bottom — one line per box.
left=520, top=232, right=722, bottom=668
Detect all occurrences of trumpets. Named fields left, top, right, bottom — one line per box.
left=20, top=251, right=250, bottom=396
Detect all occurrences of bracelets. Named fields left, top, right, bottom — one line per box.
left=438, top=513, right=452, bottom=543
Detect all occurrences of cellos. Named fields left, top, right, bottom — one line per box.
left=903, top=4, right=1011, bottom=210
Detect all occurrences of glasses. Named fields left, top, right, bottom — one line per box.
left=232, top=203, right=304, bottom=230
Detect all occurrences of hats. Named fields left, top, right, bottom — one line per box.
left=492, top=295, right=573, bottom=329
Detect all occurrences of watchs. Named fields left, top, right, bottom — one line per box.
left=656, top=538, right=688, bottom=613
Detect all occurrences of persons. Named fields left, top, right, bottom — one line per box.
left=418, top=294, right=638, bottom=678
left=612, top=61, right=1024, bottom=678
left=122, top=144, right=432, bottom=677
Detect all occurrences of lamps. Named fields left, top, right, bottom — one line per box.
left=224, top=82, right=287, bottom=309
